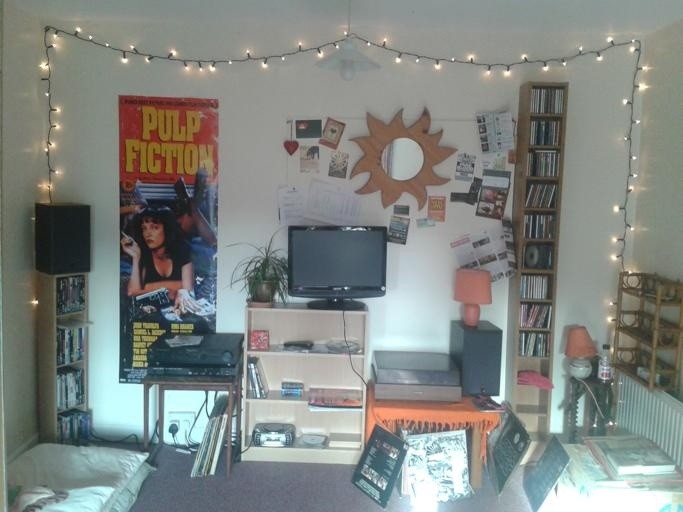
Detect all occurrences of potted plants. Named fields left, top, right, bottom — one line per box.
left=226, top=225, right=291, bottom=305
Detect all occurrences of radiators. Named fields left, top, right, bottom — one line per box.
left=613, top=363, right=683, bottom=475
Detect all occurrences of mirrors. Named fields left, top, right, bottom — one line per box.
left=347, top=105, right=460, bottom=210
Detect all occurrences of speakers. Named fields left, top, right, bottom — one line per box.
left=450, top=321, right=503, bottom=397
left=34, top=201, right=91, bottom=276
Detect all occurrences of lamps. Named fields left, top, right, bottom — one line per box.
left=564, top=324, right=598, bottom=380
left=453, top=268, right=492, bottom=327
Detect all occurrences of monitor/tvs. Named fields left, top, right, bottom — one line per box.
left=287, top=225, right=387, bottom=311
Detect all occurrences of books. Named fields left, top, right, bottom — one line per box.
left=516, top=148, right=562, bottom=358
left=530, top=85, right=564, bottom=114
left=57, top=275, right=92, bottom=446
left=307, top=387, right=363, bottom=408
left=255, top=359, right=275, bottom=398
left=248, top=358, right=256, bottom=399
left=578, top=432, right=683, bottom=492
left=251, top=358, right=262, bottom=400
left=530, top=117, right=562, bottom=145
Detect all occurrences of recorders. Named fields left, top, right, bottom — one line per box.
left=252, top=422, right=296, bottom=448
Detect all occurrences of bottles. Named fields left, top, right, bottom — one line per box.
left=596, top=344, right=613, bottom=382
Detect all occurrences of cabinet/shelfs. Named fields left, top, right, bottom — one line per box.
left=610, top=271, right=681, bottom=390
left=241, top=300, right=367, bottom=468
left=36, top=270, right=90, bottom=446
left=504, top=80, right=570, bottom=443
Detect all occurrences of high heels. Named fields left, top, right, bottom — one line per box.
left=173, top=176, right=192, bottom=210
left=193, top=168, right=209, bottom=205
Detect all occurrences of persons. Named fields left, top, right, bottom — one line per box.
left=120, top=207, right=194, bottom=301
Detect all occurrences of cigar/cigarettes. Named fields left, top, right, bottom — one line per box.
left=121, top=230, right=128, bottom=238
